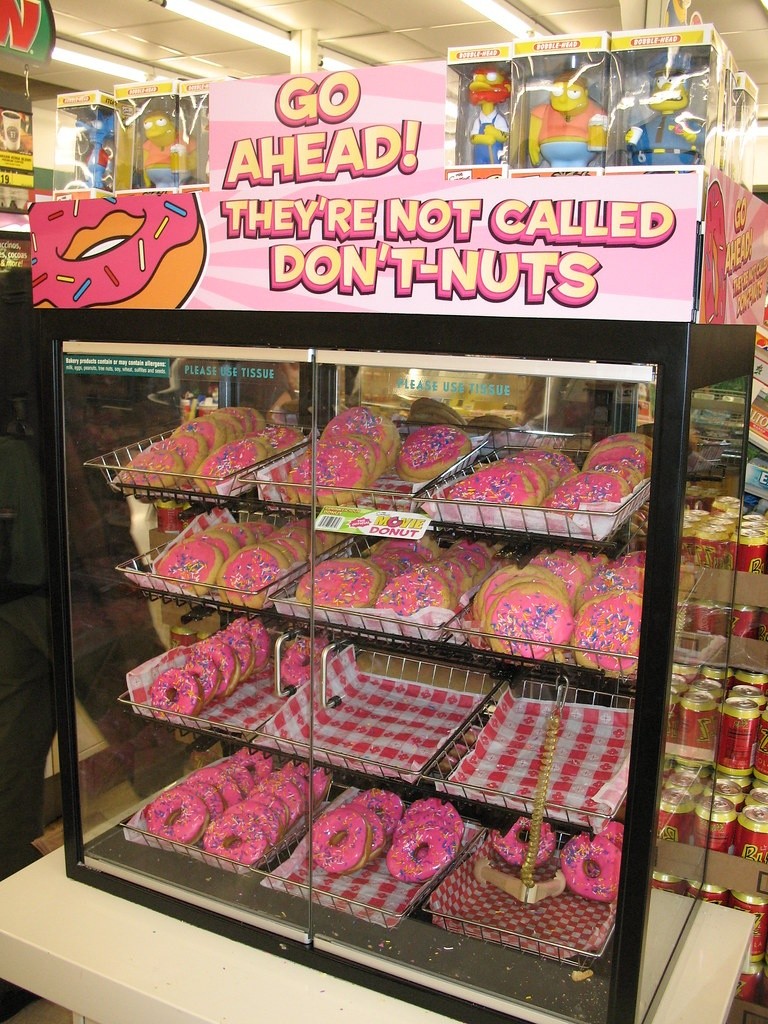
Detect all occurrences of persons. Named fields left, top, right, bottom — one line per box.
left=0, top=267, right=182, bottom=881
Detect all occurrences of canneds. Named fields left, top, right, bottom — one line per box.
left=651, top=480, right=768, bottom=1010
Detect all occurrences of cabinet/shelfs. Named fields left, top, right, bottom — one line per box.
left=89, top=424, right=648, bottom=831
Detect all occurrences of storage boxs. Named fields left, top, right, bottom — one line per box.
left=448, top=23, right=760, bottom=192
left=51, top=73, right=237, bottom=199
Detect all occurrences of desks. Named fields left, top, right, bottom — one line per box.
left=0, top=756, right=753, bottom=1024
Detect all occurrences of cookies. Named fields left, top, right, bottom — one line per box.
left=116, top=406, right=655, bottom=682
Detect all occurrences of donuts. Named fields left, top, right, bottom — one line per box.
left=146, top=746, right=626, bottom=904
left=148, top=617, right=335, bottom=719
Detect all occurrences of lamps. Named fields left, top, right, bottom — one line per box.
left=462, top=0, right=553, bottom=38
left=49, top=38, right=194, bottom=83
left=150, top=1, right=372, bottom=71
